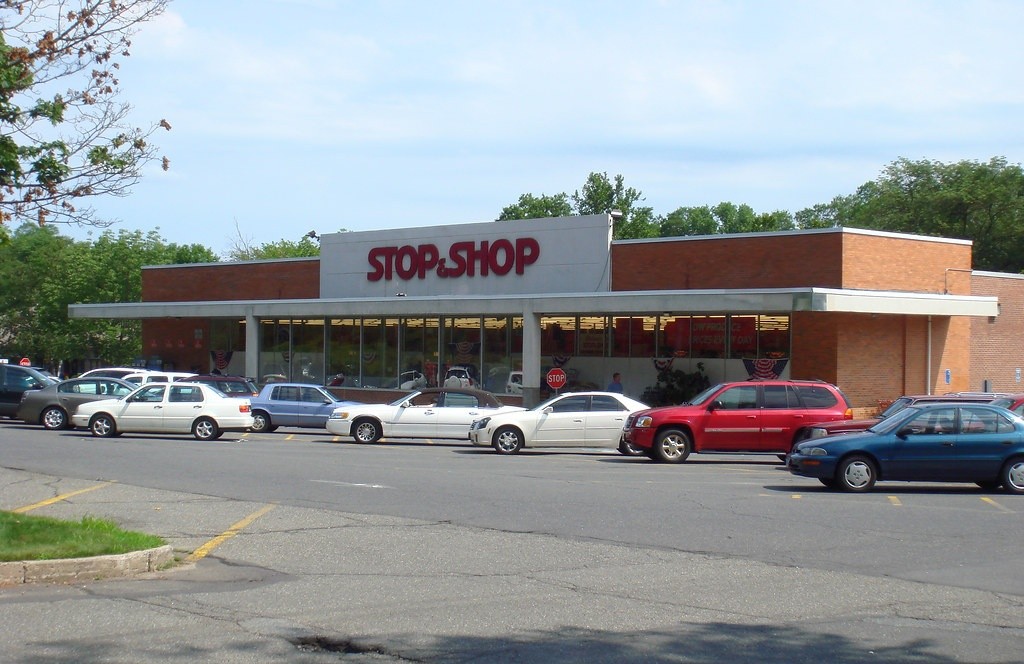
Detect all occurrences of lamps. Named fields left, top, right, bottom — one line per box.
left=663, top=311, right=673, bottom=317
left=997, top=303, right=1001, bottom=314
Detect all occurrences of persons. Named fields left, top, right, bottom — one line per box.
left=330, top=371, right=344, bottom=386
left=607, top=373, right=623, bottom=393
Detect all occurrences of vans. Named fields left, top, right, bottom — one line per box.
left=102, top=372, right=199, bottom=396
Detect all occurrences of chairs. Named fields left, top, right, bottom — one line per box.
left=100, top=386, right=105, bottom=394
left=110, top=385, right=115, bottom=396
left=940, top=417, right=953, bottom=432
left=303, top=393, right=311, bottom=402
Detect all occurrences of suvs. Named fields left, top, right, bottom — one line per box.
left=442, top=367, right=479, bottom=388
left=154, top=375, right=262, bottom=401
left=622, top=377, right=853, bottom=465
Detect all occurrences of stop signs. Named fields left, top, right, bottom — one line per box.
left=20, top=358, right=31, bottom=367
left=547, top=368, right=566, bottom=389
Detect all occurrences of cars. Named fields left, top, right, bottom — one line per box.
left=468, top=390, right=652, bottom=456
left=260, top=374, right=288, bottom=383
left=72, top=381, right=254, bottom=441
left=505, top=372, right=523, bottom=393
left=72, top=368, right=153, bottom=394
left=26, top=367, right=54, bottom=379
left=232, top=383, right=367, bottom=433
left=325, top=388, right=529, bottom=444
left=785, top=402, right=1024, bottom=492
left=921, top=393, right=1024, bottom=433
left=17, top=377, right=156, bottom=430
left=805, top=394, right=996, bottom=441
left=400, top=370, right=426, bottom=389
left=0, top=363, right=57, bottom=420
